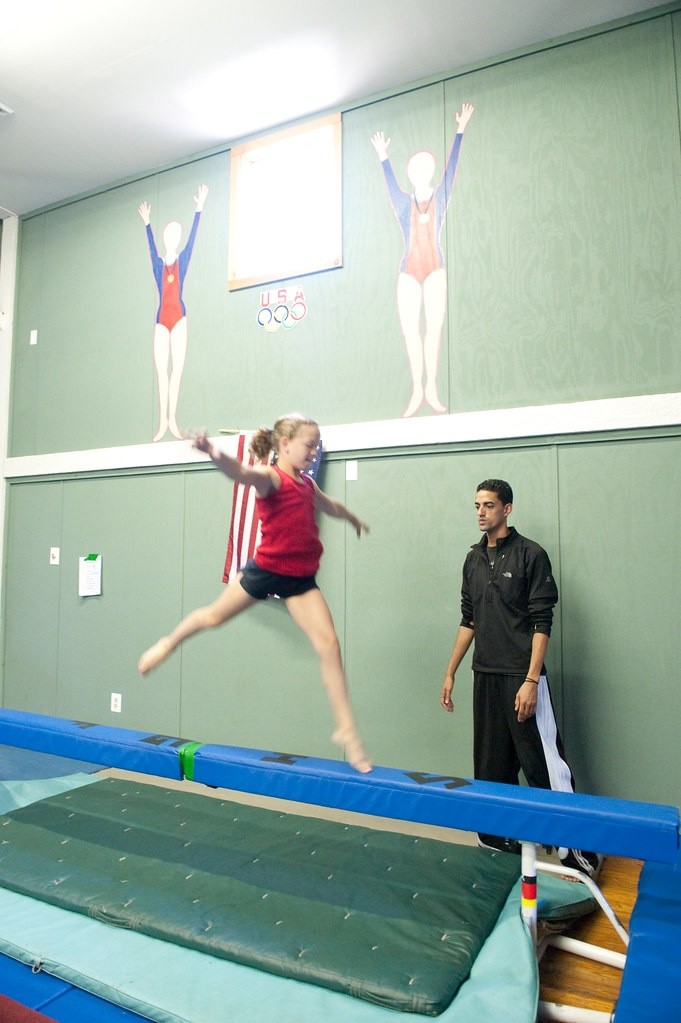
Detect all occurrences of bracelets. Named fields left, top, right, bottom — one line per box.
left=525, top=678, right=538, bottom=684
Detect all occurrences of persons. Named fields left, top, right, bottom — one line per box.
left=139, top=415, right=370, bottom=774
left=441, top=478, right=605, bottom=886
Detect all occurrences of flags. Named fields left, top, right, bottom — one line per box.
left=223, top=430, right=322, bottom=598
